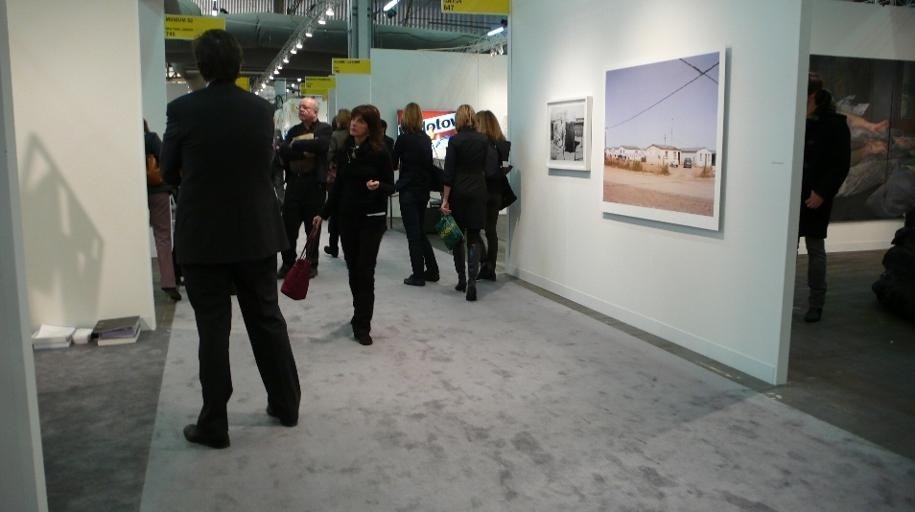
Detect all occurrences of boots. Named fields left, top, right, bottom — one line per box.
left=487, top=239, right=498, bottom=281
left=466, top=242, right=481, bottom=301
left=453, top=241, right=467, bottom=292
left=478, top=241, right=491, bottom=280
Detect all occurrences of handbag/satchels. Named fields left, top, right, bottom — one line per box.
left=147, top=154, right=162, bottom=185
left=500, top=174, right=516, bottom=210
left=434, top=215, right=465, bottom=250
left=281, top=259, right=311, bottom=300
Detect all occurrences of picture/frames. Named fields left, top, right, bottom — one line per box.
left=599, top=47, right=726, bottom=232
left=545, top=94, right=592, bottom=172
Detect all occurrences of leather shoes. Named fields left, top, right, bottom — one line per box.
left=354, top=330, right=372, bottom=345
left=184, top=425, right=230, bottom=448
left=424, top=272, right=439, bottom=281
left=277, top=265, right=287, bottom=279
left=267, top=407, right=298, bottom=426
left=324, top=246, right=338, bottom=257
left=404, top=274, right=425, bottom=286
left=309, top=266, right=317, bottom=278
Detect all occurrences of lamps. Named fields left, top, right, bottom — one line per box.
left=272, top=3, right=335, bottom=75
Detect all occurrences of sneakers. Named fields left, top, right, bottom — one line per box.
left=162, top=287, right=181, bottom=301
left=429, top=166, right=444, bottom=191
left=803, top=307, right=824, bottom=325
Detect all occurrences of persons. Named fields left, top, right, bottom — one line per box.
left=323, top=109, right=350, bottom=260
left=272, top=113, right=284, bottom=215
left=392, top=101, right=447, bottom=289
left=159, top=26, right=304, bottom=450
left=796, top=71, right=854, bottom=336
left=143, top=115, right=187, bottom=303
left=275, top=91, right=325, bottom=280
left=378, top=115, right=398, bottom=234
left=309, top=103, right=396, bottom=347
left=472, top=108, right=517, bottom=285
left=439, top=103, right=490, bottom=302
